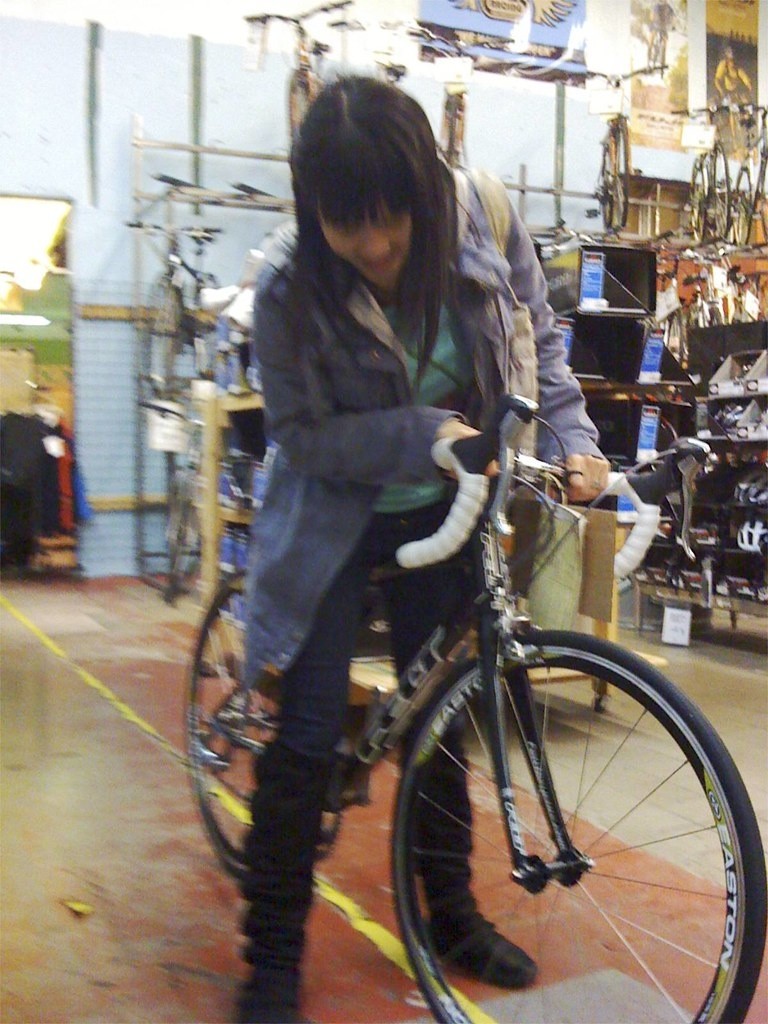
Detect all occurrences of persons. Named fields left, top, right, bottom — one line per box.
left=237, top=76, right=611, bottom=1024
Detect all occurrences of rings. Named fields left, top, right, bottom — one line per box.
left=591, top=481, right=600, bottom=488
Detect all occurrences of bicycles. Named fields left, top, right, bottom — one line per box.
left=632, top=102, right=768, bottom=368
left=570, top=65, right=670, bottom=231
left=174, top=393, right=768, bottom=1024
left=123, top=175, right=292, bottom=606
left=239, top=0, right=355, bottom=147
left=406, top=23, right=516, bottom=168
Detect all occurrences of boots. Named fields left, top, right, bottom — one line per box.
left=236, top=741, right=332, bottom=1024
left=404, top=760, right=538, bottom=988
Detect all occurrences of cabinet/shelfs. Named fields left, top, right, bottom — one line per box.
left=201, top=396, right=627, bottom=718
left=625, top=376, right=768, bottom=638
